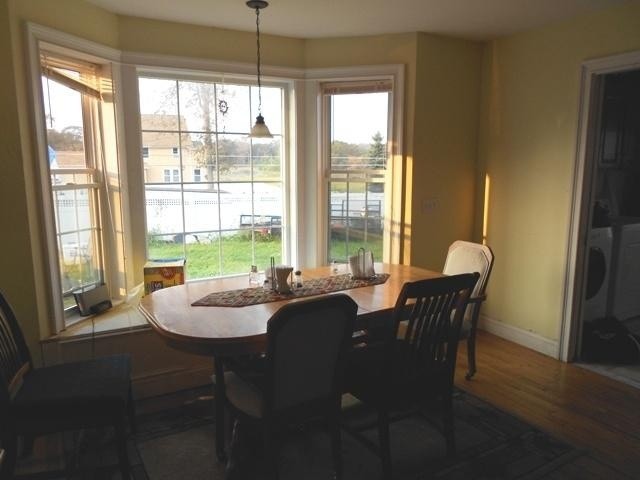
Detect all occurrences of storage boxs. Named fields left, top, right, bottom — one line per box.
left=143, top=258, right=186, bottom=295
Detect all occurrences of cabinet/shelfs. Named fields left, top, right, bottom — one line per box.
left=601, top=86, right=640, bottom=170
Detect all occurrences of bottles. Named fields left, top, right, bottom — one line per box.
left=332, top=260, right=338, bottom=273
left=249, top=265, right=271, bottom=292
left=294, top=271, right=304, bottom=289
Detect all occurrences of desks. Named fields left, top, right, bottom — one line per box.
left=144, top=265, right=447, bottom=480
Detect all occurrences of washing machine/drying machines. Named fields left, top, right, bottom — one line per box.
left=582, top=226, right=614, bottom=325
left=615, top=223, right=640, bottom=323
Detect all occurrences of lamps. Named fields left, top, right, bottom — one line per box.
left=247, top=0, right=272, bottom=141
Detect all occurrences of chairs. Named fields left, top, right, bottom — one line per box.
left=0, top=289, right=141, bottom=477
left=214, top=295, right=356, bottom=480
left=349, top=270, right=482, bottom=478
left=441, top=240, right=495, bottom=381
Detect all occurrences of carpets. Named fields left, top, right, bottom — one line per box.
left=78, top=384, right=640, bottom=479
